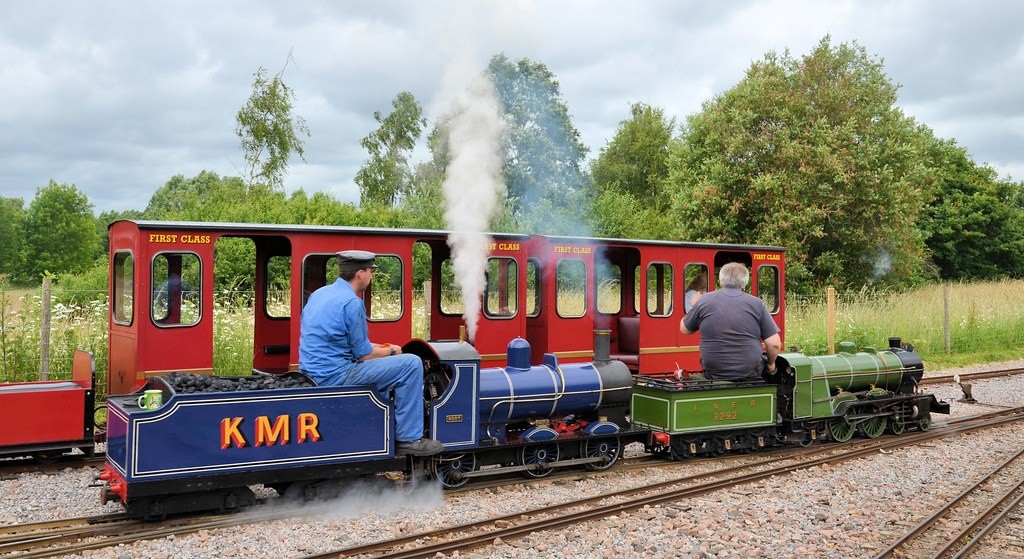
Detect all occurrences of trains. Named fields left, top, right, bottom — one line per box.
left=0, top=217, right=788, bottom=464
left=85, top=337, right=953, bottom=523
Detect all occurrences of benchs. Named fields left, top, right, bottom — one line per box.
left=608, top=317, right=639, bottom=365
left=251, top=366, right=288, bottom=377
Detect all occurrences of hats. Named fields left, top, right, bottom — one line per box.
left=336, top=251, right=378, bottom=271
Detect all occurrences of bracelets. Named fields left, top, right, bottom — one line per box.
left=388, top=345, right=397, bottom=355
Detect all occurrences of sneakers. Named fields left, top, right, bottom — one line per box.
left=396, top=437, right=443, bottom=456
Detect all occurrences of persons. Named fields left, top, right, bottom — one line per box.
left=666, top=273, right=707, bottom=315
left=678, top=262, right=782, bottom=381
left=298, top=250, right=444, bottom=458
